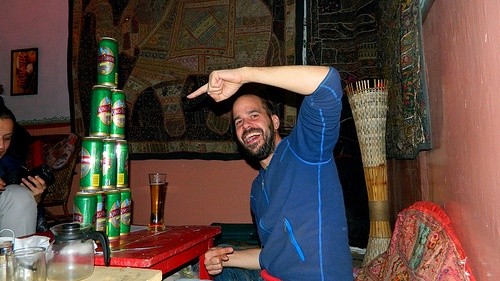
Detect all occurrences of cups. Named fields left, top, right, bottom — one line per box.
left=5, top=247, right=46, bottom=281
left=148, top=173, right=169, bottom=230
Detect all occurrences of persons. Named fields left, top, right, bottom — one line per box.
left=186, top=65, right=355, bottom=281
left=0, top=96, right=47, bottom=237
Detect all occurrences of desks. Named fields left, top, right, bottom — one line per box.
left=47, top=224, right=222, bottom=281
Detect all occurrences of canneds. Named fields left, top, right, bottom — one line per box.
left=109, top=89, right=126, bottom=139
left=73, top=188, right=131, bottom=243
left=96, top=37, right=118, bottom=89
left=79, top=137, right=130, bottom=190
left=88, top=85, right=112, bottom=137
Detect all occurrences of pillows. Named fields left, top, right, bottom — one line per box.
left=353, top=202, right=476, bottom=281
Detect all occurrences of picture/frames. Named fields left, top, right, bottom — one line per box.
left=9, top=47, right=39, bottom=97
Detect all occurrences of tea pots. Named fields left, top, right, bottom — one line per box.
left=45, top=221, right=110, bottom=281
